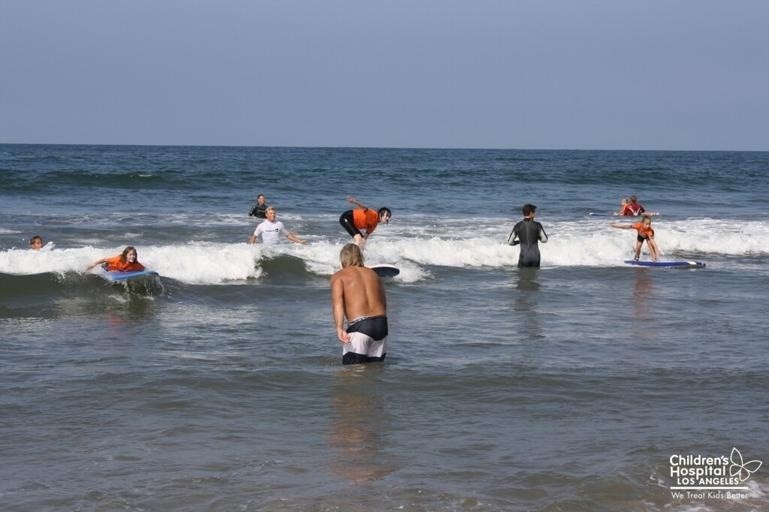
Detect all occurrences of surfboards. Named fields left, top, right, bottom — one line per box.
left=370, top=263, right=400, bottom=276
left=110, top=270, right=159, bottom=282
left=624, top=260, right=707, bottom=267
left=590, top=211, right=659, bottom=217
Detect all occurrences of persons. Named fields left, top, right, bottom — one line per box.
left=247, top=206, right=310, bottom=245
left=623, top=196, right=646, bottom=217
left=338, top=195, right=392, bottom=253
left=617, top=198, right=628, bottom=216
left=508, top=204, right=548, bottom=269
left=79, top=246, right=147, bottom=275
left=29, top=235, right=45, bottom=250
left=249, top=194, right=269, bottom=219
left=611, top=214, right=664, bottom=264
left=331, top=243, right=389, bottom=364
